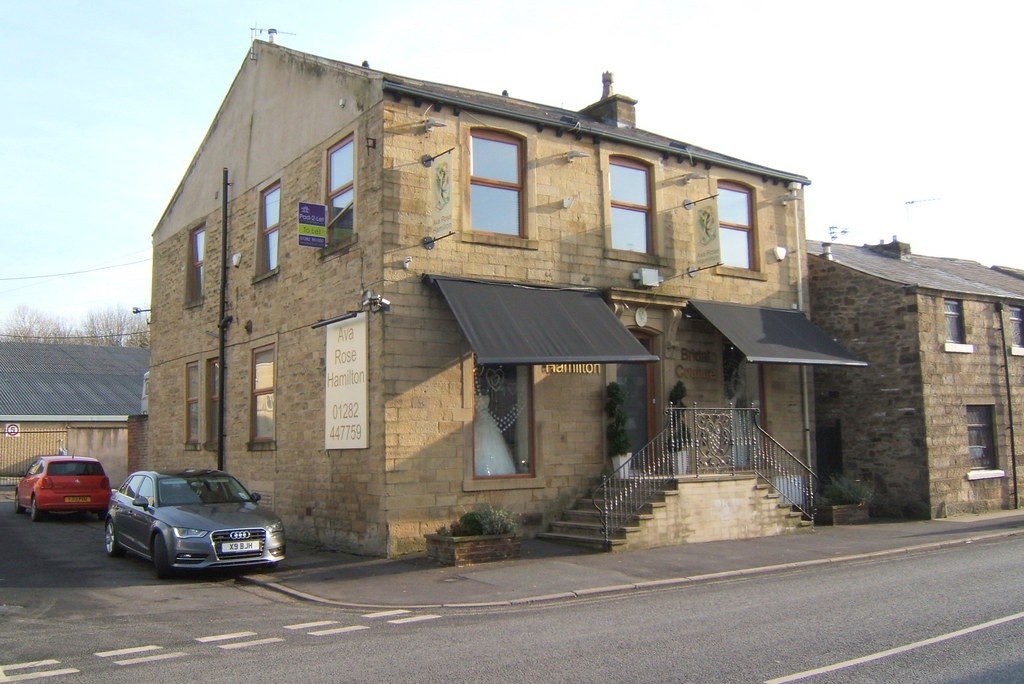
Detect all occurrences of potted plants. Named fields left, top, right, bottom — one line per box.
left=666, top=380, right=691, bottom=474
left=802, top=469, right=888, bottom=524
left=603, top=381, right=633, bottom=479
left=423, top=503, right=522, bottom=566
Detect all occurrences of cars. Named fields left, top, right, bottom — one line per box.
left=103, top=468, right=287, bottom=580
left=14, top=455, right=112, bottom=522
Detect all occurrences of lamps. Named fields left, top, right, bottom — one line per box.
left=425, top=116, right=447, bottom=133
left=782, top=194, right=802, bottom=206
left=567, top=149, right=590, bottom=162
left=683, top=172, right=706, bottom=184
